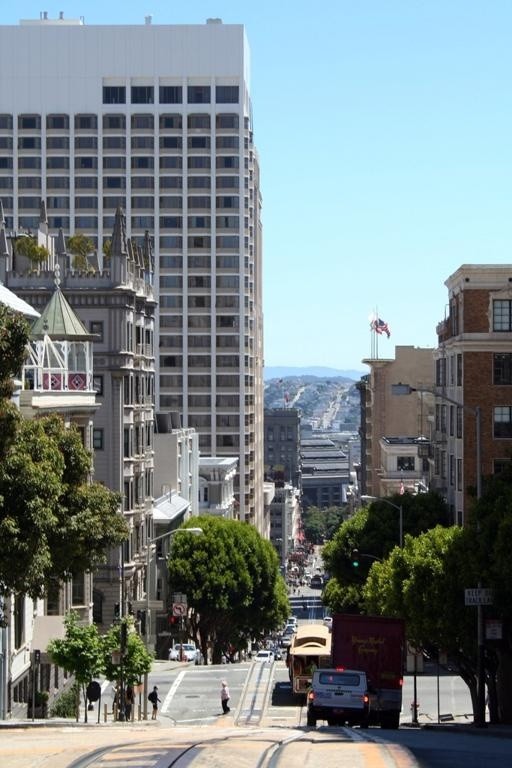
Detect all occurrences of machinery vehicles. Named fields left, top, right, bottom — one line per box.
left=286, top=618, right=331, bottom=701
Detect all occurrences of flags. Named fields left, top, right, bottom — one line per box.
left=399, top=481, right=405, bottom=496
left=370, top=318, right=391, bottom=340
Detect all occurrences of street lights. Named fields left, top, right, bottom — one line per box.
left=143, top=527, right=203, bottom=722
left=391, top=382, right=484, bottom=724
left=359, top=495, right=417, bottom=725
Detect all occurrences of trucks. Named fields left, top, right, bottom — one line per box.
left=325, top=613, right=407, bottom=730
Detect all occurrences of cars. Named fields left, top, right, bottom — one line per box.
left=253, top=539, right=331, bottom=664
left=167, top=643, right=202, bottom=665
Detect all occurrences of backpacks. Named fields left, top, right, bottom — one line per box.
left=147, top=692, right=155, bottom=701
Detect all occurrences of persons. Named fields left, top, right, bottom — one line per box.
left=220, top=681, right=231, bottom=715
left=284, top=540, right=317, bottom=595
left=150, top=686, right=162, bottom=720
left=247, top=629, right=282, bottom=663
left=111, top=685, right=135, bottom=722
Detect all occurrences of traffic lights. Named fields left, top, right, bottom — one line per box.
left=352, top=548, right=360, bottom=577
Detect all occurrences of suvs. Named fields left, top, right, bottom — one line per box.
left=306, top=668, right=371, bottom=729
